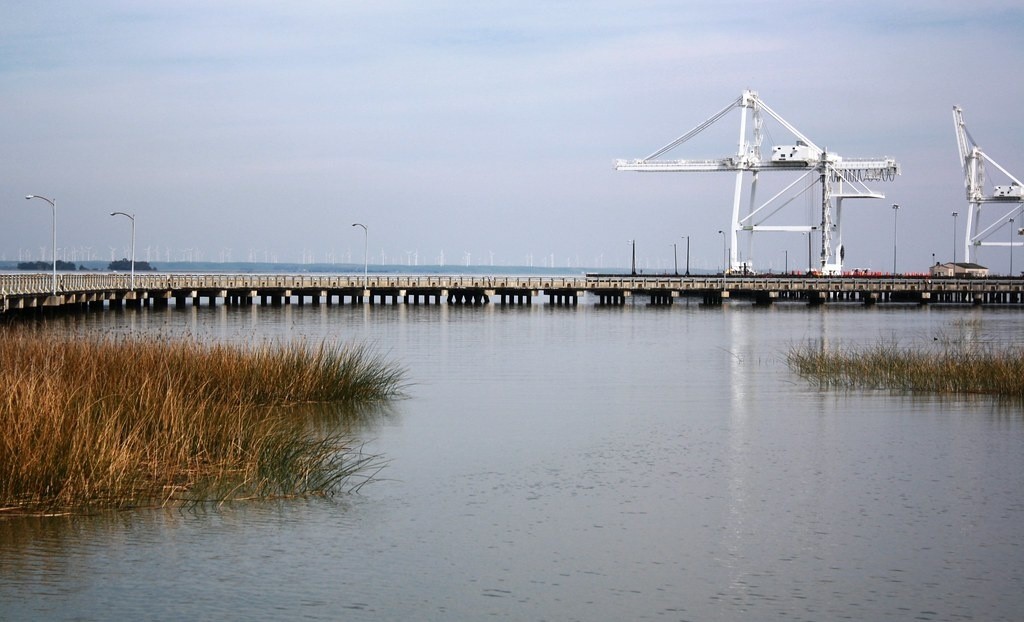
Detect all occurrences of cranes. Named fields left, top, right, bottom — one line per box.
left=611, top=88, right=903, bottom=278
left=951, top=104, right=1024, bottom=264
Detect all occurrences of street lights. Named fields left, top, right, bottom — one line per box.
left=24, top=195, right=57, bottom=297
left=890, top=203, right=900, bottom=276
left=351, top=223, right=368, bottom=290
left=951, top=211, right=958, bottom=278
left=1007, top=217, right=1014, bottom=280
left=110, top=212, right=135, bottom=292
